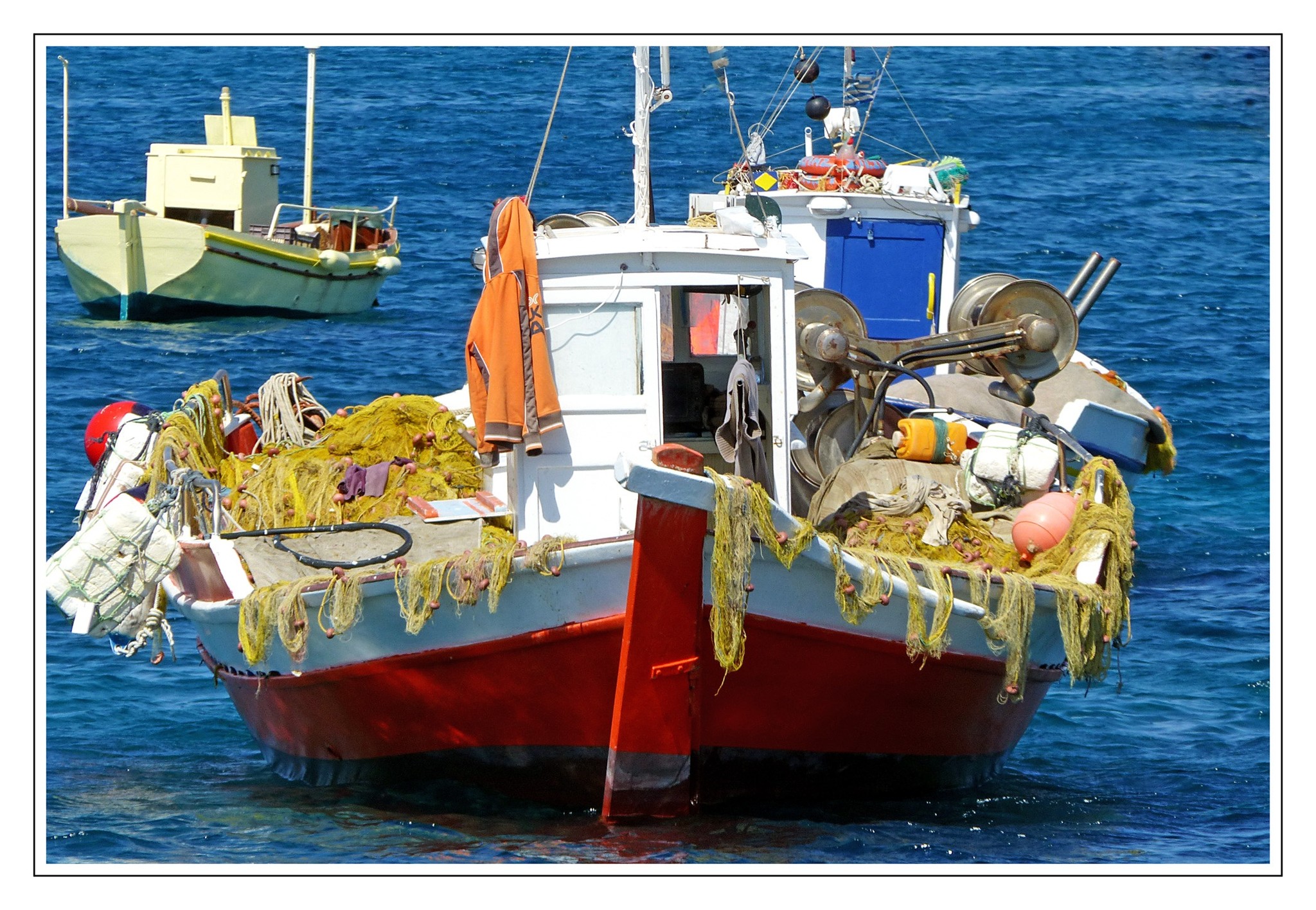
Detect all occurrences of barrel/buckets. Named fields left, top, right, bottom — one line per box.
left=892, top=419, right=967, bottom=461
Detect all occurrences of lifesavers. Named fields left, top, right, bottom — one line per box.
left=797, top=156, right=886, bottom=192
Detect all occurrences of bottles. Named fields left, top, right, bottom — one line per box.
left=838, top=138, right=865, bottom=160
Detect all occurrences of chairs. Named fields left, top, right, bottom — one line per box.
left=662, top=361, right=704, bottom=438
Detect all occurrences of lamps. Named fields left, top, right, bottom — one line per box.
left=807, top=196, right=851, bottom=221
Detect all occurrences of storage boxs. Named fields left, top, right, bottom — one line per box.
left=296, top=233, right=318, bottom=250
left=249, top=223, right=295, bottom=244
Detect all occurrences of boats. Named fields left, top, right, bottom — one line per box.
left=43, top=36, right=1158, bottom=831
left=53, top=85, right=402, bottom=315
